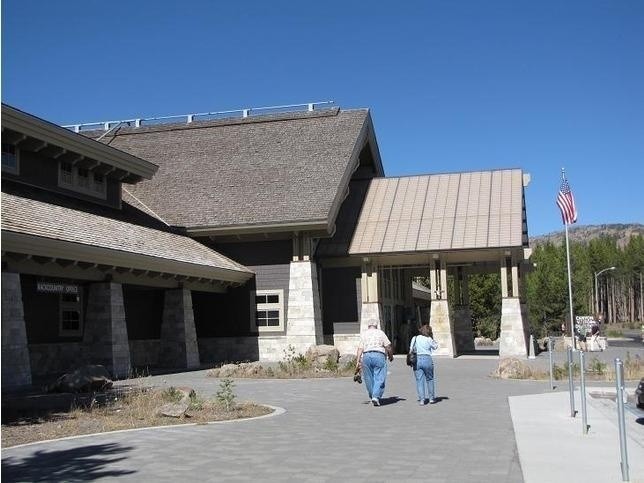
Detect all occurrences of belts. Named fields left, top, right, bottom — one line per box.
left=363, top=350, right=384, bottom=353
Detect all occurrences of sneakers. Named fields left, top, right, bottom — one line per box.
left=371, top=397, right=380, bottom=405
left=419, top=398, right=425, bottom=405
left=429, top=398, right=437, bottom=403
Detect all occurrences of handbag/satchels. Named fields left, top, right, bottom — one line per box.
left=407, top=335, right=418, bottom=366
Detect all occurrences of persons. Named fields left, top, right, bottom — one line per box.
left=354, top=319, right=394, bottom=406
left=409, top=324, right=438, bottom=406
left=592, top=323, right=600, bottom=337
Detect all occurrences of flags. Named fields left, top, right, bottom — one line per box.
left=556, top=177, right=577, bottom=224
